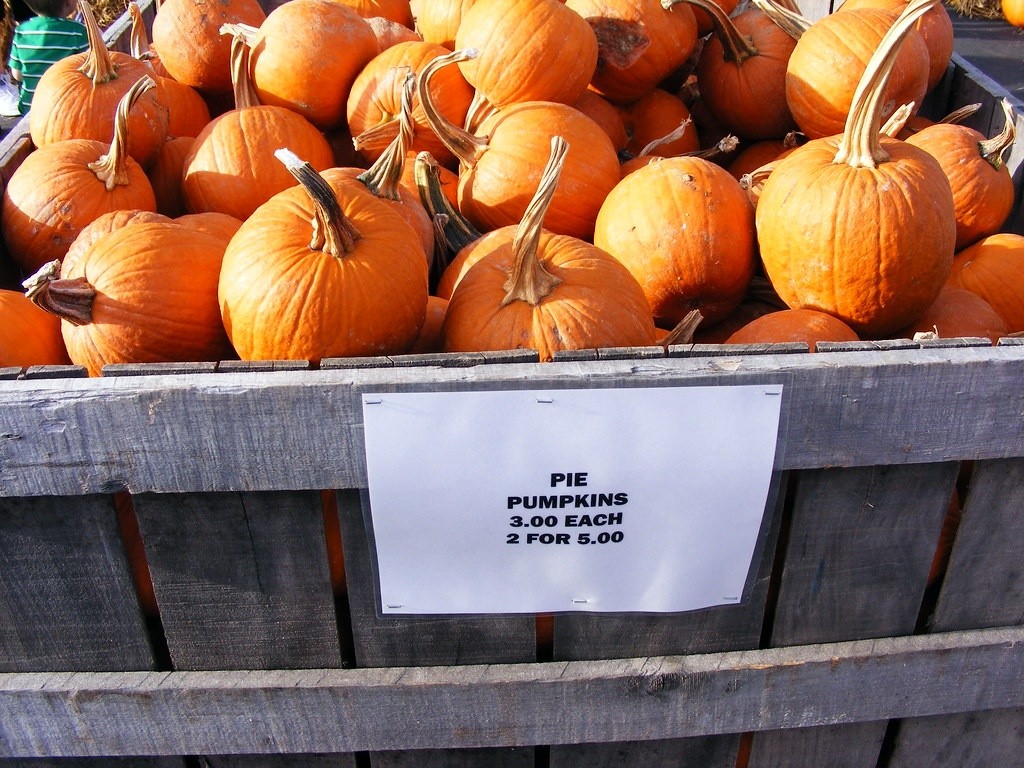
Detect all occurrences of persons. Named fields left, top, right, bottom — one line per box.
left=8, top=0, right=90, bottom=118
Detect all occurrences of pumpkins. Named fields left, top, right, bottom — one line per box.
left=0, top=0, right=1024, bottom=645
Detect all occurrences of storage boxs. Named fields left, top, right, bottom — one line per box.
left=0, top=0, right=1024, bottom=768
